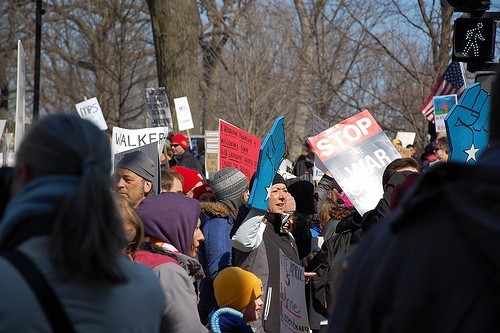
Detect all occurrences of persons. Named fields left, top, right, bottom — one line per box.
left=0, top=72, right=500, bottom=333
left=126, top=192, right=210, bottom=333
left=204, top=266, right=263, bottom=333
left=229, top=170, right=317, bottom=333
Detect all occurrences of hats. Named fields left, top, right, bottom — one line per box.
left=281, top=192, right=296, bottom=212
left=336, top=191, right=354, bottom=207
left=170, top=165, right=204, bottom=193
left=116, top=151, right=157, bottom=182
left=248, top=171, right=287, bottom=196
left=213, top=167, right=248, bottom=215
left=171, top=134, right=189, bottom=149
left=214, top=267, right=263, bottom=309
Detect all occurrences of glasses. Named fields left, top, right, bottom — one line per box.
left=170, top=143, right=178, bottom=148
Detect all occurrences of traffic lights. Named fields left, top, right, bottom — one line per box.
left=451, top=17, right=498, bottom=62
